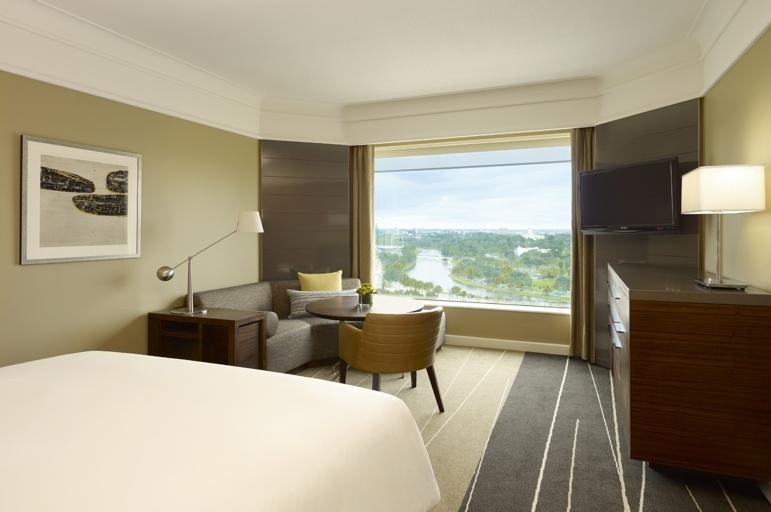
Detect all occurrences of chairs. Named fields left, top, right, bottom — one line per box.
left=336, top=305, right=444, bottom=413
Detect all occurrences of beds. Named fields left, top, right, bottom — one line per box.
left=0, top=350, right=442, bottom=511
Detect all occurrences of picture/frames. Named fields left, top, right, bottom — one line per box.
left=20, top=132, right=142, bottom=265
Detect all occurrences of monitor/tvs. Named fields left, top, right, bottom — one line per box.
left=579, top=156, right=681, bottom=236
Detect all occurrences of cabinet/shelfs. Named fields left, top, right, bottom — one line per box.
left=606, top=264, right=769, bottom=488
left=146, top=306, right=268, bottom=370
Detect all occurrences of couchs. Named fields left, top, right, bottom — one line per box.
left=184, top=278, right=371, bottom=374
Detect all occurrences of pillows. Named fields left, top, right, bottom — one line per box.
left=286, top=268, right=357, bottom=319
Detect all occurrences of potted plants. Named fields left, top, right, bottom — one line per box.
left=356, top=281, right=375, bottom=304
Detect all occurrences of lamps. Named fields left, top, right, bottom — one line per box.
left=157, top=212, right=266, bottom=314
left=679, top=165, right=766, bottom=291
